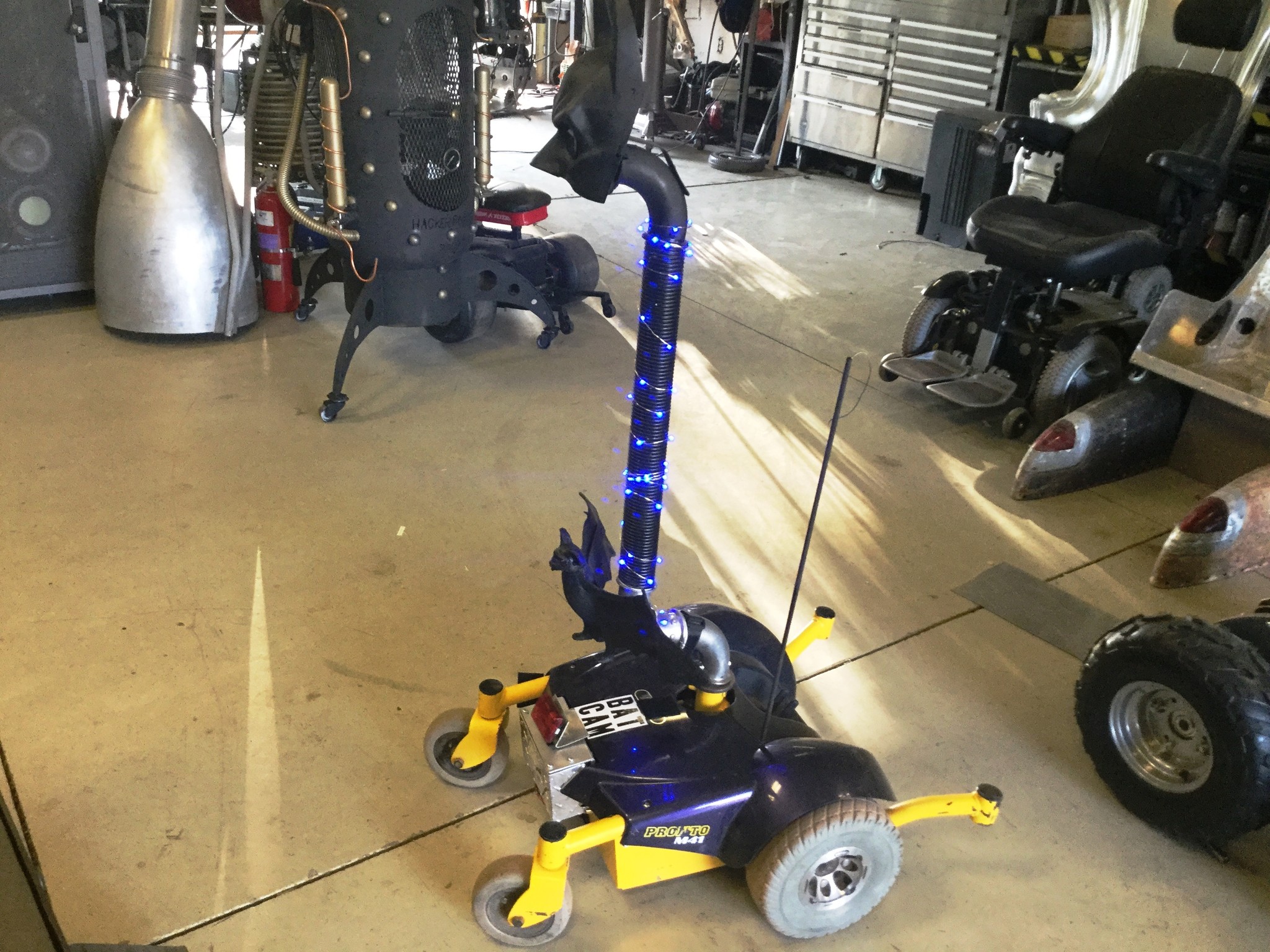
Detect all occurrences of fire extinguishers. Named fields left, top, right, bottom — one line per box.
left=253, top=161, right=303, bottom=314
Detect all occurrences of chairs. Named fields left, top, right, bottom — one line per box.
left=929, top=66, right=1254, bottom=410
